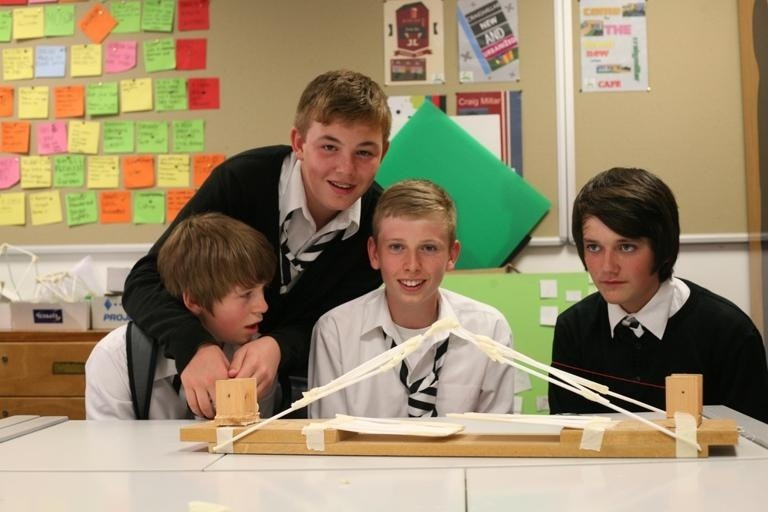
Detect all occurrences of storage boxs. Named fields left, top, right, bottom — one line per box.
left=10, top=300, right=91, bottom=332
left=92, top=297, right=133, bottom=334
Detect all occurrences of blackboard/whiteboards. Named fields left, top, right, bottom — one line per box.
left=0, top=0, right=569, bottom=255
left=564, top=0, right=768, bottom=245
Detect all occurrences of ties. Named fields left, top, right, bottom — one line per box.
left=280, top=212, right=347, bottom=286
left=622, top=319, right=646, bottom=338
left=382, top=329, right=450, bottom=417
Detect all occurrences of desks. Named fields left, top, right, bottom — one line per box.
left=0, top=405, right=767, bottom=512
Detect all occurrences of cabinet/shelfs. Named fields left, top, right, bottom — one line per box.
left=1, top=341, right=98, bottom=419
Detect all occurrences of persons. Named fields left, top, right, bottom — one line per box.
left=548, top=167, right=767, bottom=423
left=84, top=212, right=283, bottom=420
left=121, top=70, right=392, bottom=419
left=306, top=180, right=514, bottom=418
